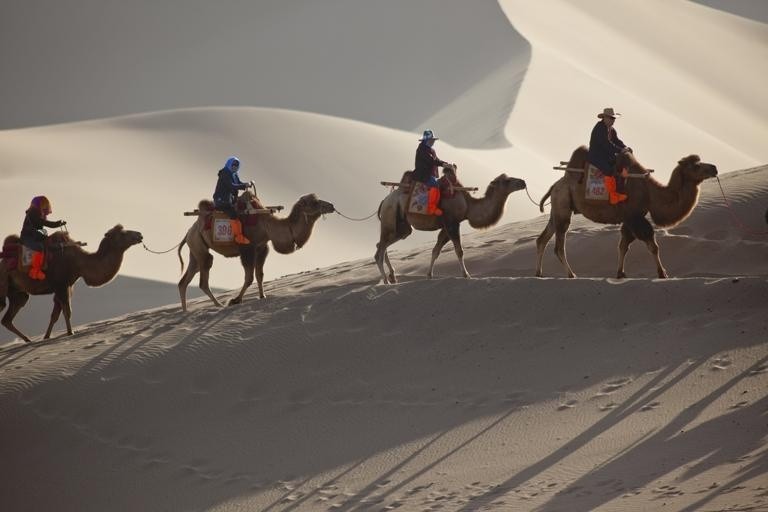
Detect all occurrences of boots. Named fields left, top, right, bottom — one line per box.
left=230, top=219, right=250, bottom=245
left=604, top=176, right=627, bottom=205
left=29, top=251, right=46, bottom=280
left=426, top=187, right=443, bottom=216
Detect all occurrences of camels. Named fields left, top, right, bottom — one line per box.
left=375, top=164, right=525, bottom=285
left=536, top=145, right=717, bottom=279
left=178, top=191, right=334, bottom=312
left=0, top=224, right=143, bottom=342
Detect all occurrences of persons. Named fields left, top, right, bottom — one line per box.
left=213, top=156, right=252, bottom=244
left=588, top=109, right=630, bottom=205
left=19, top=196, right=66, bottom=280
left=413, top=130, right=456, bottom=216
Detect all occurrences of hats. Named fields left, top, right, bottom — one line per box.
left=419, top=130, right=439, bottom=141
left=598, top=108, right=622, bottom=119
